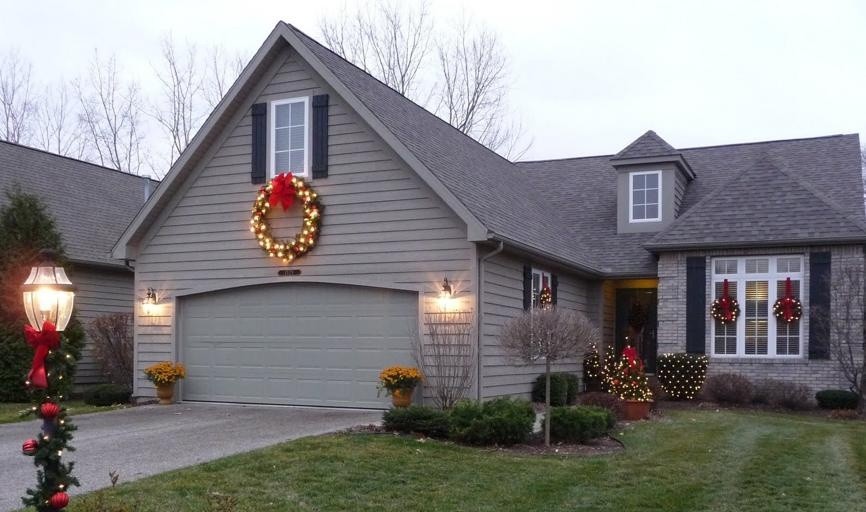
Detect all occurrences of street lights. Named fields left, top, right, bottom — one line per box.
left=19, top=246, right=78, bottom=512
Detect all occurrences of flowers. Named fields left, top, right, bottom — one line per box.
left=143, top=359, right=186, bottom=386
left=376, top=366, right=424, bottom=398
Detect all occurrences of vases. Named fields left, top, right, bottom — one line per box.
left=624, top=399, right=655, bottom=421
left=153, top=382, right=176, bottom=404
left=391, top=388, right=413, bottom=406
